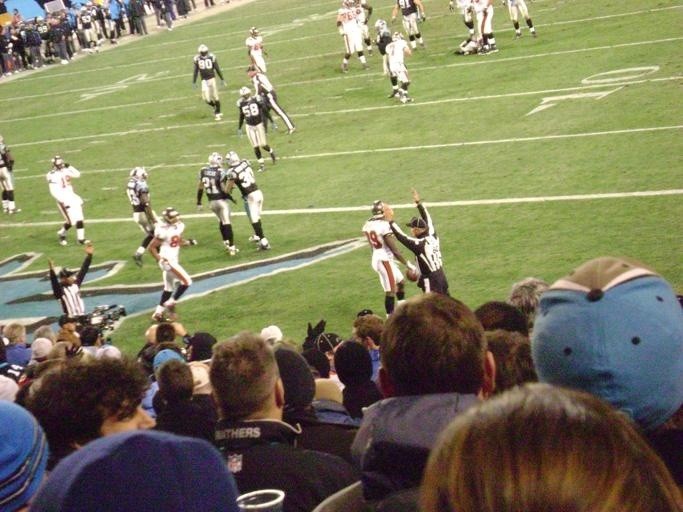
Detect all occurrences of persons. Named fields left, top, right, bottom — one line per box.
left=126, top=166, right=157, bottom=268
left=337, top=2, right=371, bottom=74
left=192, top=44, right=228, bottom=121
left=247, top=65, right=297, bottom=137
left=311, top=295, right=498, bottom=510
left=192, top=333, right=357, bottom=512
left=149, top=205, right=198, bottom=321
left=36, top=355, right=156, bottom=452
left=376, top=18, right=392, bottom=77
left=386, top=32, right=412, bottom=103
left=45, top=155, right=86, bottom=247
left=392, top=0, right=427, bottom=52
left=45, top=239, right=96, bottom=321
left=0, top=133, right=24, bottom=215
left=1, top=274, right=568, bottom=414
left=245, top=26, right=270, bottom=78
left=348, top=0, right=373, bottom=57
left=220, top=151, right=270, bottom=251
left=419, top=381, right=680, bottom=511
left=380, top=184, right=449, bottom=300
left=0, top=0, right=223, bottom=76
left=236, top=84, right=278, bottom=174
left=362, top=200, right=417, bottom=321
left=450, top=0, right=499, bottom=56
left=503, top=0, right=536, bottom=40
left=198, top=151, right=240, bottom=257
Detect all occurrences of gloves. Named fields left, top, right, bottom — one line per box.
left=339, top=29, right=346, bottom=36
left=305, top=318, right=328, bottom=346
left=65, top=343, right=86, bottom=361
left=237, top=129, right=243, bottom=139
left=192, top=84, right=198, bottom=90
left=158, top=258, right=171, bottom=272
left=220, top=80, right=227, bottom=88
left=196, top=203, right=204, bottom=213
left=185, top=239, right=197, bottom=246
left=272, top=123, right=278, bottom=134
left=63, top=203, right=70, bottom=210
left=420, top=13, right=427, bottom=22
left=406, top=260, right=419, bottom=271
left=391, top=16, right=396, bottom=24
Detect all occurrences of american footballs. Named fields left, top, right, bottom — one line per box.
left=407, top=268, right=420, bottom=282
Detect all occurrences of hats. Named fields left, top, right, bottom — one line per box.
left=260, top=325, right=282, bottom=342
left=246, top=64, right=257, bottom=71
left=313, top=331, right=343, bottom=352
left=31, top=337, right=53, bottom=358
left=273, top=348, right=317, bottom=410
left=333, top=340, right=374, bottom=383
left=96, top=345, right=121, bottom=361
left=405, top=216, right=427, bottom=229
left=1, top=375, right=21, bottom=396
left=152, top=349, right=184, bottom=372
left=59, top=267, right=77, bottom=278
left=0, top=359, right=27, bottom=380
left=184, top=332, right=217, bottom=348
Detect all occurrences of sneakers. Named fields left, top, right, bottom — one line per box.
left=132, top=251, right=143, bottom=268
left=249, top=235, right=260, bottom=242
left=399, top=95, right=415, bottom=104
left=257, top=165, right=265, bottom=173
left=229, top=246, right=236, bottom=256
left=514, top=34, right=523, bottom=40
left=368, top=50, right=375, bottom=56
left=490, top=43, right=499, bottom=52
left=341, top=63, right=350, bottom=74
left=361, top=62, right=372, bottom=70
left=288, top=127, right=296, bottom=136
left=3, top=208, right=9, bottom=213
left=166, top=301, right=178, bottom=320
left=151, top=311, right=166, bottom=323
left=9, top=209, right=21, bottom=213
left=531, top=31, right=537, bottom=38
left=76, top=238, right=91, bottom=245
left=257, top=238, right=272, bottom=251
left=225, top=245, right=239, bottom=253
left=56, top=231, right=67, bottom=246
left=272, top=155, right=276, bottom=165
left=388, top=92, right=401, bottom=98
left=478, top=47, right=490, bottom=55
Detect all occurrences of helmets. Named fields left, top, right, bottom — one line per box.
left=375, top=19, right=387, bottom=33
left=392, top=32, right=401, bottom=41
left=249, top=26, right=260, bottom=37
left=51, top=155, right=65, bottom=169
left=239, top=86, right=251, bottom=96
left=129, top=166, right=148, bottom=180
left=162, top=207, right=180, bottom=224
left=198, top=44, right=209, bottom=52
left=225, top=151, right=241, bottom=167
left=372, top=199, right=385, bottom=215
left=207, top=152, right=223, bottom=168
left=343, top=0, right=354, bottom=7
